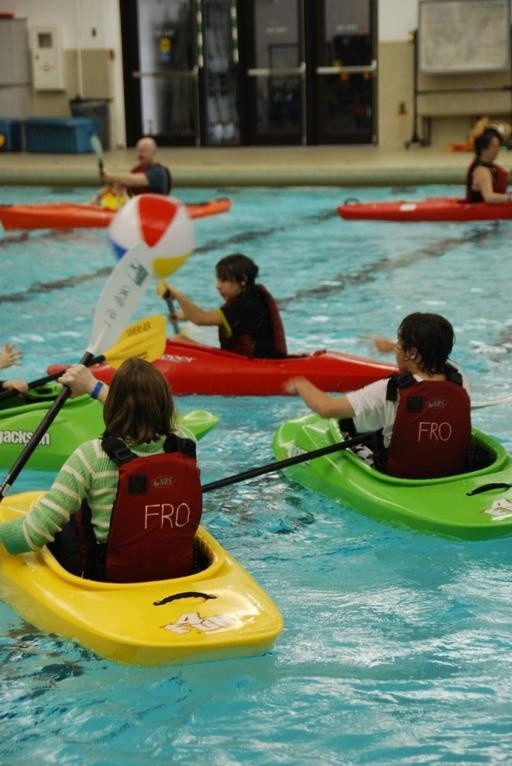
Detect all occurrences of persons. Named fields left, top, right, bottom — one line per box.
left=0, top=342, right=29, bottom=394
left=98, top=137, right=172, bottom=199
left=0, top=357, right=203, bottom=582
left=158, top=254, right=286, bottom=359
left=285, top=314, right=470, bottom=477
left=467, top=131, right=512, bottom=204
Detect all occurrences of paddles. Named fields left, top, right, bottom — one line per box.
left=0, top=315, right=166, bottom=401
left=90, top=135, right=104, bottom=178
left=204, top=393, right=512, bottom=495
left=0, top=241, right=156, bottom=505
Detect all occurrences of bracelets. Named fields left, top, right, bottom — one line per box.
left=90, top=382, right=103, bottom=399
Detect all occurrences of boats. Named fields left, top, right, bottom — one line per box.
left=338, top=194, right=512, bottom=223
left=0, top=196, right=232, bottom=233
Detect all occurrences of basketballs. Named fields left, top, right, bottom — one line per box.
left=108, top=195, right=195, bottom=277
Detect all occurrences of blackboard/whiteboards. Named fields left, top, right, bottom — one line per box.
left=418, top=0, right=510, bottom=74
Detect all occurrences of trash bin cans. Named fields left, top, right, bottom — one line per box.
left=71, top=99, right=109, bottom=151
